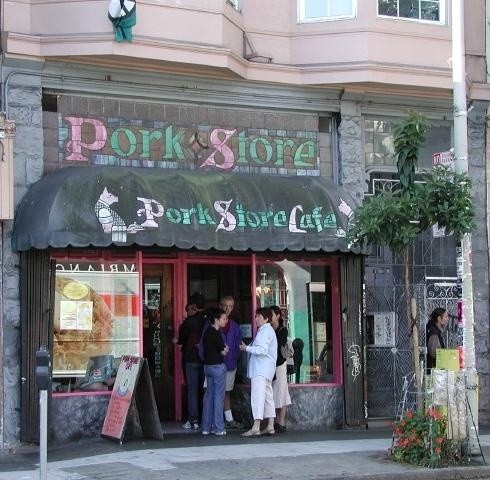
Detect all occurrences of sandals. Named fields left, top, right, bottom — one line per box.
left=240, top=428, right=261, bottom=437
left=261, top=426, right=276, bottom=435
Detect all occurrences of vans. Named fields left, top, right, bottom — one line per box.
left=309, top=281, right=328, bottom=368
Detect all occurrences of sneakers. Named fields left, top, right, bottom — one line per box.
left=181, top=419, right=244, bottom=436
left=274, top=422, right=287, bottom=434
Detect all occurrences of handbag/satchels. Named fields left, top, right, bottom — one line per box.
left=281, top=337, right=295, bottom=359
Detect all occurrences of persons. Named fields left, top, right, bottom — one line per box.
left=198, top=295, right=246, bottom=428
left=271, top=306, right=292, bottom=433
left=174, top=304, right=196, bottom=423
left=427, top=307, right=451, bottom=374
left=202, top=310, right=230, bottom=435
left=177, top=292, right=207, bottom=430
left=239, top=308, right=278, bottom=437
